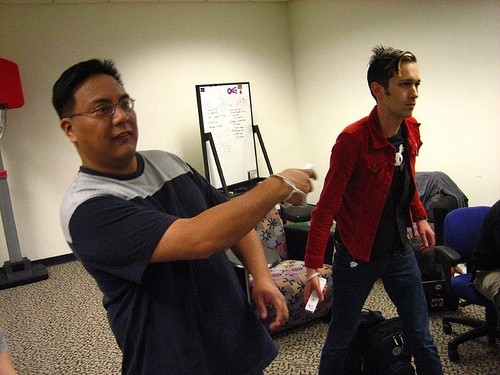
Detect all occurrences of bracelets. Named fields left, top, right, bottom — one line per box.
left=303, top=271, right=321, bottom=286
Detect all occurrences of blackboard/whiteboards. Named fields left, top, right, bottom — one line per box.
left=194, top=80, right=259, bottom=191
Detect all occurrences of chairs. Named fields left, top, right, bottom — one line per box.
left=218, top=185, right=334, bottom=336
left=434, top=206, right=500, bottom=363
left=408, top=170, right=467, bottom=306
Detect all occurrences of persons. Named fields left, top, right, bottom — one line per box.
left=304, top=47, right=445, bottom=375
left=52, top=58, right=318, bottom=375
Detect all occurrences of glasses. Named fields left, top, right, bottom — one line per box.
left=67, top=97, right=136, bottom=119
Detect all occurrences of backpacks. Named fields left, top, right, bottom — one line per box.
left=346, top=308, right=417, bottom=375
